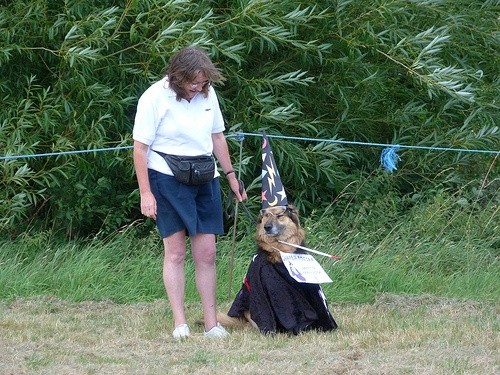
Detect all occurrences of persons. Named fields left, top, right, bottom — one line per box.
left=132, top=47, right=247, bottom=338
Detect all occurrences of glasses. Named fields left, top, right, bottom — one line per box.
left=189, top=80, right=209, bottom=87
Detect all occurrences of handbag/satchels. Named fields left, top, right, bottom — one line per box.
left=153, top=150, right=214, bottom=185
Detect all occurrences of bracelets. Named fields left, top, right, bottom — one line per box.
left=224, top=169, right=234, bottom=176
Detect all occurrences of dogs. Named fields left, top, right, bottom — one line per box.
left=195, top=205, right=328, bottom=332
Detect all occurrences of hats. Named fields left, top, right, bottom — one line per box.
left=260, top=129, right=289, bottom=210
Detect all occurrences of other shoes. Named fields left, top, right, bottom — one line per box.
left=172, top=324, right=191, bottom=338
left=204, top=321, right=231, bottom=338
